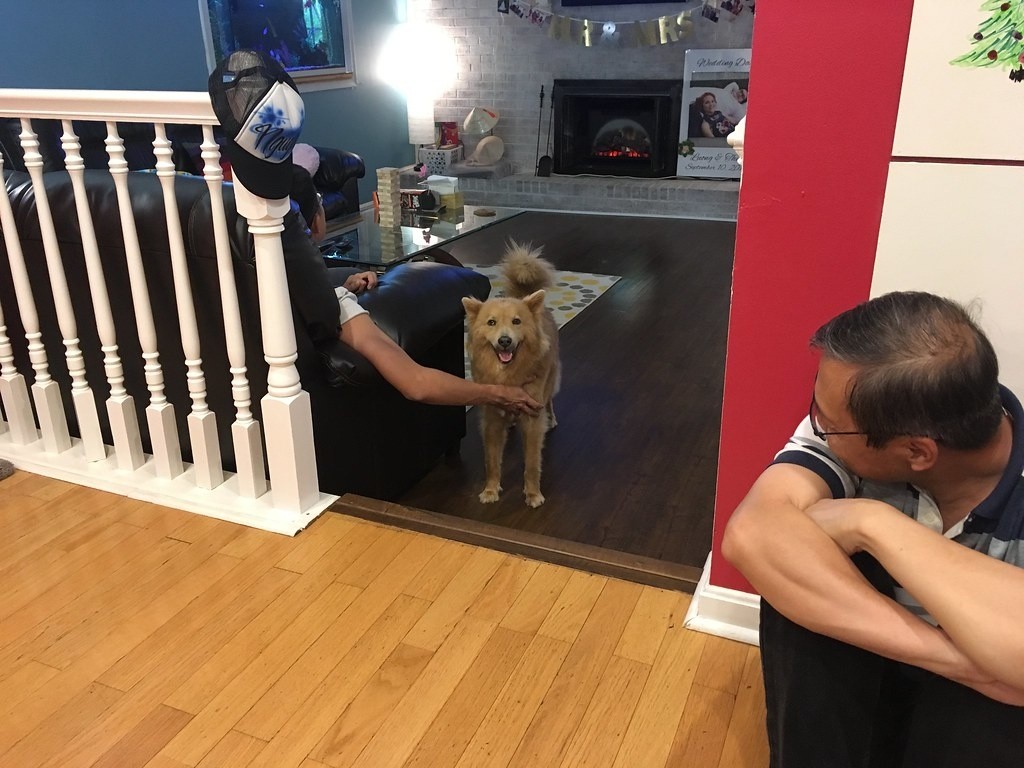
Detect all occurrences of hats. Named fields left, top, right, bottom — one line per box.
left=208, top=50, right=306, bottom=200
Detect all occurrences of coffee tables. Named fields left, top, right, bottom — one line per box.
left=317, top=204, right=528, bottom=269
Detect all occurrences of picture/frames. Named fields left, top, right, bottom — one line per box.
left=199, top=0, right=358, bottom=95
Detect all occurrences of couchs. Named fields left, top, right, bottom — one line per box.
left=0, top=114, right=493, bottom=507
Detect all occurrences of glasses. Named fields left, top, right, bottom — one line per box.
left=810, top=371, right=943, bottom=443
left=310, top=192, right=323, bottom=226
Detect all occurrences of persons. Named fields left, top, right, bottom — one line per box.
left=289, top=163, right=544, bottom=418
left=719, top=290, right=1024, bottom=768
left=689, top=81, right=747, bottom=124
left=700, top=92, right=738, bottom=138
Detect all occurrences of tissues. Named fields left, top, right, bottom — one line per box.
left=417, top=173, right=466, bottom=209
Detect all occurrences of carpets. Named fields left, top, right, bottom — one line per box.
left=444, top=264, right=622, bottom=411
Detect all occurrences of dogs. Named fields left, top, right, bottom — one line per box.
left=461, top=238, right=562, bottom=508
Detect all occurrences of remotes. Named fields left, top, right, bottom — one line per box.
left=335, top=238, right=352, bottom=249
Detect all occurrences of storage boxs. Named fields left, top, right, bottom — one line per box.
left=416, top=145, right=464, bottom=177
